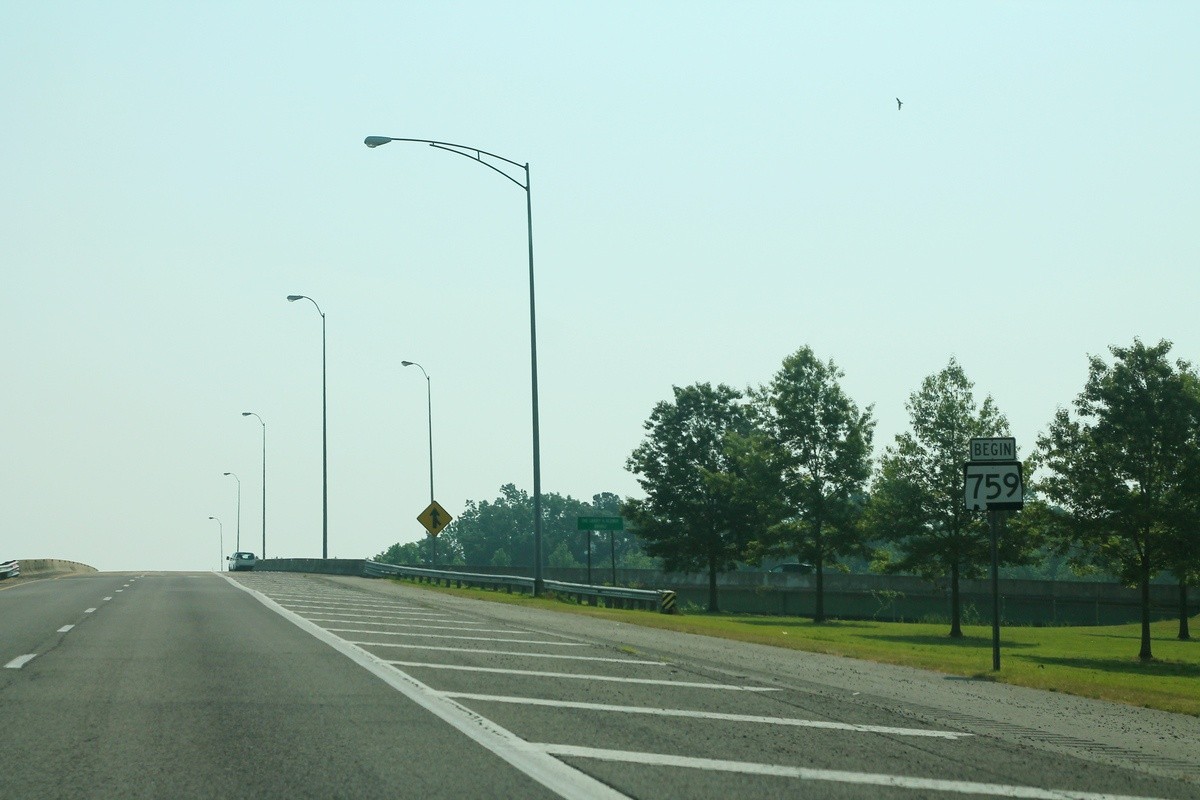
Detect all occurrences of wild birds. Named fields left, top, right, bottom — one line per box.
left=896, top=97, right=904, bottom=110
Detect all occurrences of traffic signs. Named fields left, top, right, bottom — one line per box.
left=577, top=517, right=623, bottom=529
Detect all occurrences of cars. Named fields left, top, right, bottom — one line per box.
left=768, top=564, right=815, bottom=574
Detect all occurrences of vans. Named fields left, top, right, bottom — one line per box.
left=225, top=552, right=256, bottom=571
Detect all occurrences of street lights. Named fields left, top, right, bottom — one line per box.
left=209, top=517, right=223, bottom=571
left=365, top=135, right=544, bottom=599
left=224, top=472, right=240, bottom=552
left=287, top=294, right=328, bottom=559
left=241, top=412, right=266, bottom=559
left=401, top=360, right=437, bottom=568
